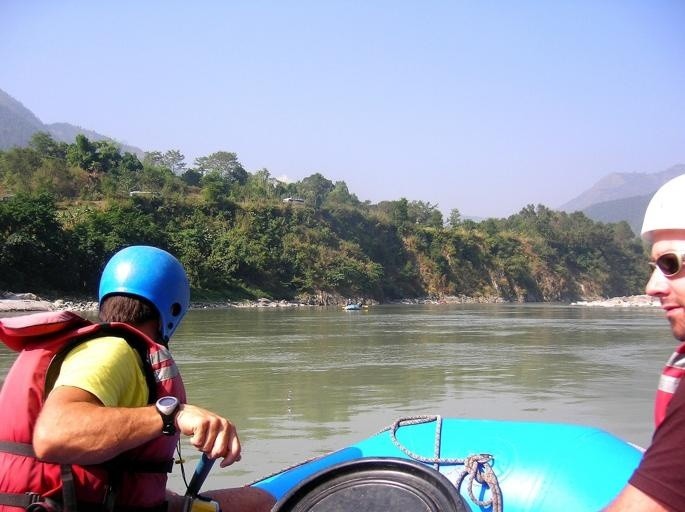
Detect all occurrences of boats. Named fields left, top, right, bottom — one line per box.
left=345, top=304, right=360, bottom=311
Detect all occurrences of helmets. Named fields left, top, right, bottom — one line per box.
left=640, top=173, right=684, bottom=244
left=97, top=245, right=190, bottom=339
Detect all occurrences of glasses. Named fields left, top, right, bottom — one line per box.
left=648, top=255, right=685, bottom=277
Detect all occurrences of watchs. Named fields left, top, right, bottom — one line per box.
left=156, top=395, right=179, bottom=437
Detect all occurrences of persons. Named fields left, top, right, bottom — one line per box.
left=596, top=170, right=685, bottom=512
left=0, top=245, right=242, bottom=512
left=348, top=299, right=352, bottom=305
left=357, top=301, right=362, bottom=306
